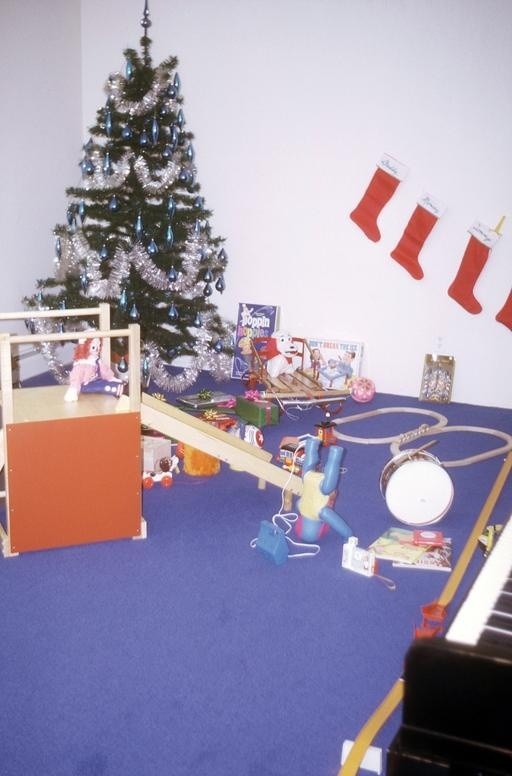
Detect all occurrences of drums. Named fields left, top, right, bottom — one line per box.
left=379, top=438, right=455, bottom=526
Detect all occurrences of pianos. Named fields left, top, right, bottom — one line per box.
left=380, top=516, right=511, bottom=776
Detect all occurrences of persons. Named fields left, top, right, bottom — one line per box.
left=64, top=328, right=124, bottom=403
